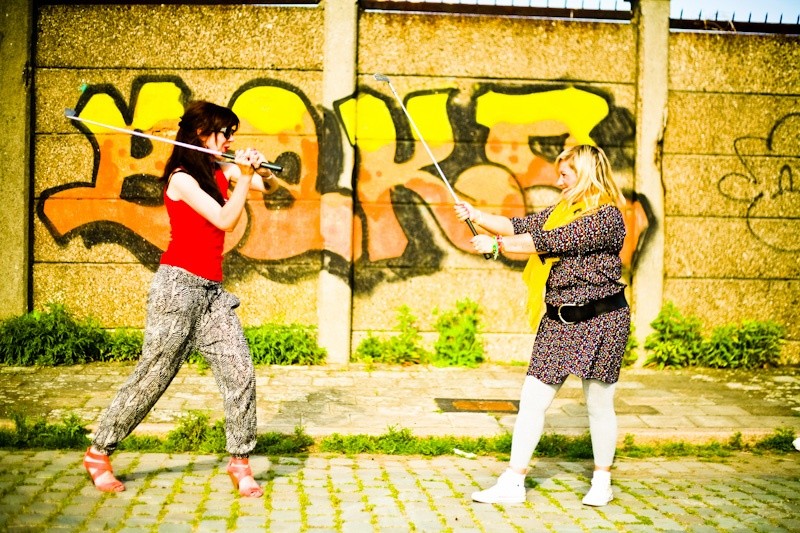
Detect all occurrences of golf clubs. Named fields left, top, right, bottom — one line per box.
left=372, top=74, right=492, bottom=260
left=64, top=108, right=285, bottom=174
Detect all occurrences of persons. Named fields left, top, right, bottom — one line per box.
left=455, top=145, right=631, bottom=507
left=83, top=100, right=279, bottom=497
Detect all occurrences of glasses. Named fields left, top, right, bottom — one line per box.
left=210, top=128, right=231, bottom=140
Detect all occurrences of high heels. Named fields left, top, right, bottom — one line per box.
left=83, top=446, right=125, bottom=493
left=229, top=456, right=263, bottom=498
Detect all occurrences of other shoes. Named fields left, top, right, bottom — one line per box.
left=582, top=477, right=613, bottom=506
left=472, top=480, right=527, bottom=504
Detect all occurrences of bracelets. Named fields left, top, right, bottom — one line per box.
left=492, top=234, right=503, bottom=260
left=265, top=174, right=273, bottom=180
